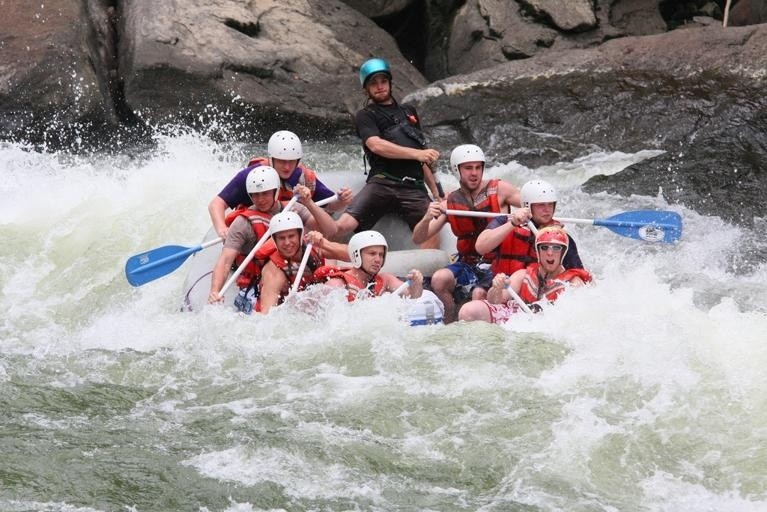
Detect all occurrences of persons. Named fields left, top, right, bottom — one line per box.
left=474, top=178, right=584, bottom=277
left=207, top=165, right=338, bottom=305
left=208, top=130, right=354, bottom=240
left=335, top=58, right=449, bottom=249
left=458, top=226, right=586, bottom=325
left=323, top=229, right=424, bottom=303
left=259, top=210, right=351, bottom=315
left=411, top=143, right=522, bottom=324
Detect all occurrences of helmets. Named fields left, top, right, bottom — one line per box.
left=450, top=144, right=484, bottom=182
left=358, top=58, right=392, bottom=88
left=518, top=180, right=556, bottom=215
left=347, top=230, right=387, bottom=269
left=534, top=226, right=569, bottom=265
left=245, top=166, right=280, bottom=203
left=268, top=131, right=302, bottom=168
left=269, top=211, right=304, bottom=250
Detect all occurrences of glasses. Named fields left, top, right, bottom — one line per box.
left=538, top=245, right=562, bottom=252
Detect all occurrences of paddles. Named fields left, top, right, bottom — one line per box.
left=444, top=209, right=683, bottom=245
left=123, top=196, right=342, bottom=288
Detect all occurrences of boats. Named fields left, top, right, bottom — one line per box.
left=179, top=170, right=477, bottom=328
